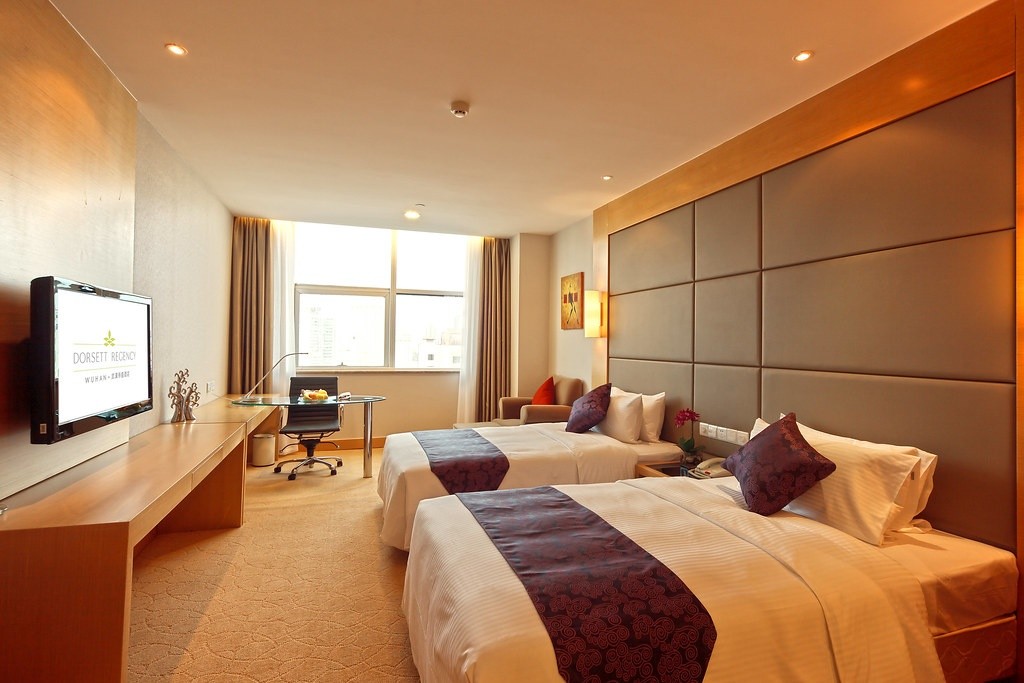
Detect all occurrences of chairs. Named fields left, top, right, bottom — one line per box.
left=273, top=375, right=344, bottom=480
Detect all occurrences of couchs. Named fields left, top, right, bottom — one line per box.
left=451, top=374, right=583, bottom=429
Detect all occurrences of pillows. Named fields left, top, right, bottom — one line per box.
left=592, top=386, right=643, bottom=445
left=750, top=418, right=922, bottom=548
left=638, top=391, right=666, bottom=443
left=720, top=411, right=837, bottom=517
left=530, top=376, right=556, bottom=405
left=780, top=412, right=939, bottom=536
left=565, top=382, right=612, bottom=434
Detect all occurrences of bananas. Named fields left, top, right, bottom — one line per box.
left=304, top=391, right=312, bottom=399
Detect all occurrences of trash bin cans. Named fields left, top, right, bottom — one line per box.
left=253, top=433, right=275, bottom=466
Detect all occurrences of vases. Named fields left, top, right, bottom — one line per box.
left=685, top=452, right=702, bottom=463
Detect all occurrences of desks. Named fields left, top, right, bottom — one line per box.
left=163, top=392, right=279, bottom=526
left=232, top=394, right=386, bottom=479
left=0, top=422, right=247, bottom=683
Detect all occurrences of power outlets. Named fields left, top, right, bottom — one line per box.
left=211, top=380, right=215, bottom=390
left=717, top=426, right=727, bottom=442
left=207, top=382, right=212, bottom=392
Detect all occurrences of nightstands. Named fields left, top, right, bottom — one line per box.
left=634, top=460, right=698, bottom=479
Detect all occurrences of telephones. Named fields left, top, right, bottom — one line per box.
left=694, top=458, right=730, bottom=478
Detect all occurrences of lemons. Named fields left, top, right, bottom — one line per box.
left=315, top=389, right=328, bottom=400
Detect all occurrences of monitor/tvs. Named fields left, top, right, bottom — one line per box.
left=19, top=277, right=154, bottom=445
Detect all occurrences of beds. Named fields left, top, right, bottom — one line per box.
left=396, top=477, right=1018, bottom=683
left=378, top=421, right=683, bottom=553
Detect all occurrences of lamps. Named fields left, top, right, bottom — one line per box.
left=583, top=290, right=608, bottom=339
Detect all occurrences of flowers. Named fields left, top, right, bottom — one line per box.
left=673, top=407, right=706, bottom=453
